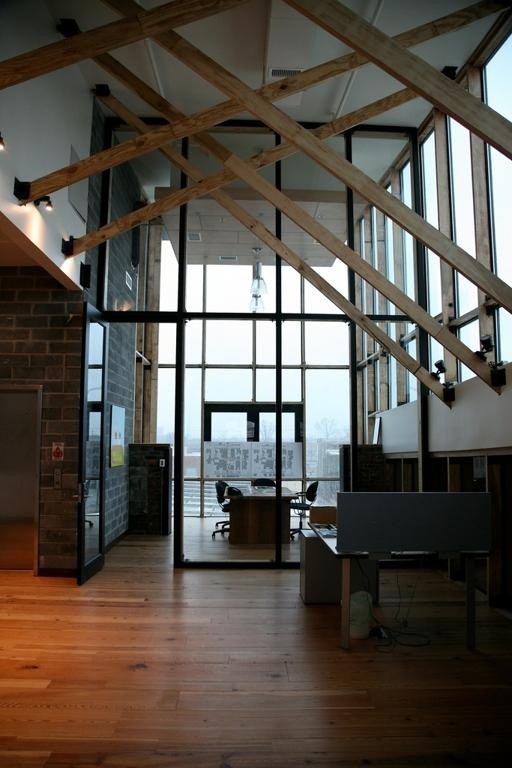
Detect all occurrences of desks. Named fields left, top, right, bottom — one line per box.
left=309, top=522, right=489, bottom=650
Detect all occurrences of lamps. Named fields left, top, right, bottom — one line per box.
left=35, top=196, right=53, bottom=212
left=431, top=360, right=447, bottom=381
left=0, top=132, right=5, bottom=151
left=475, top=335, right=493, bottom=361
left=248, top=248, right=268, bottom=311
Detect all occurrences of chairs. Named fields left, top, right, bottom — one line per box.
left=212, top=478, right=318, bottom=544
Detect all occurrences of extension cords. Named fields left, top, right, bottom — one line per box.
left=380, top=627, right=388, bottom=639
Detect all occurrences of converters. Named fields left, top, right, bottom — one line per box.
left=369, top=626, right=381, bottom=640
